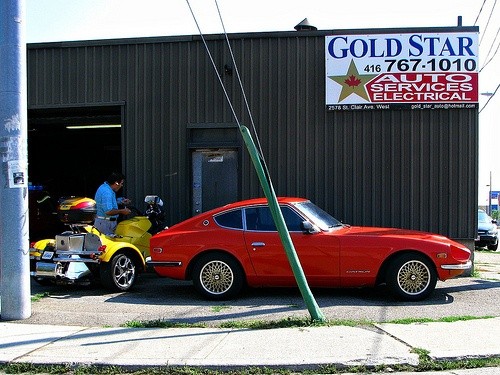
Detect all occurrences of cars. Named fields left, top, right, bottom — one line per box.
left=475, top=209, right=499, bottom=251
left=151, top=197, right=473, bottom=301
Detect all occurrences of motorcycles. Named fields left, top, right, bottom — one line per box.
left=28, top=196, right=168, bottom=292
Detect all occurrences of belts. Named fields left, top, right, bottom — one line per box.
left=97, top=216, right=117, bottom=220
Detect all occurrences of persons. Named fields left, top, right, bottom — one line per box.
left=93, top=173, right=133, bottom=238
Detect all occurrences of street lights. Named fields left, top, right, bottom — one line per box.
left=486, top=185, right=491, bottom=216
